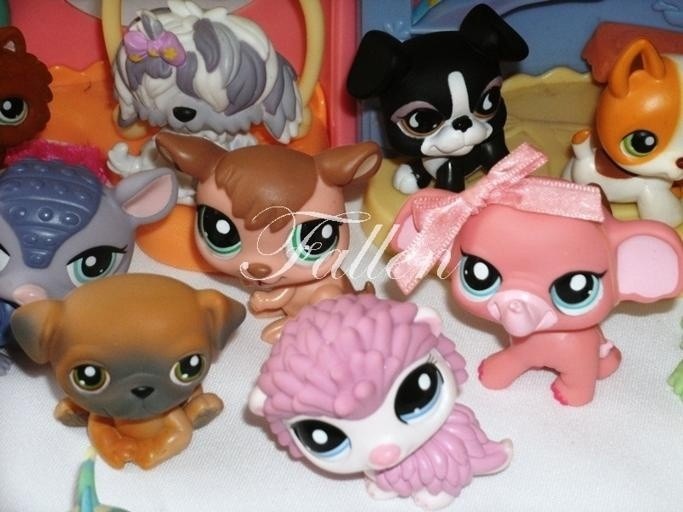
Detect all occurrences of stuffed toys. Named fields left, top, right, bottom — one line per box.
left=344, top=3, right=530, bottom=195
left=0, top=25, right=52, bottom=158
left=388, top=141, right=683, bottom=407
left=559, top=38, right=683, bottom=229
left=246, top=293, right=514, bottom=511
left=156, top=133, right=383, bottom=343
left=9, top=273, right=245, bottom=470
left=105, top=0, right=306, bottom=208
left=0, top=155, right=180, bottom=377
